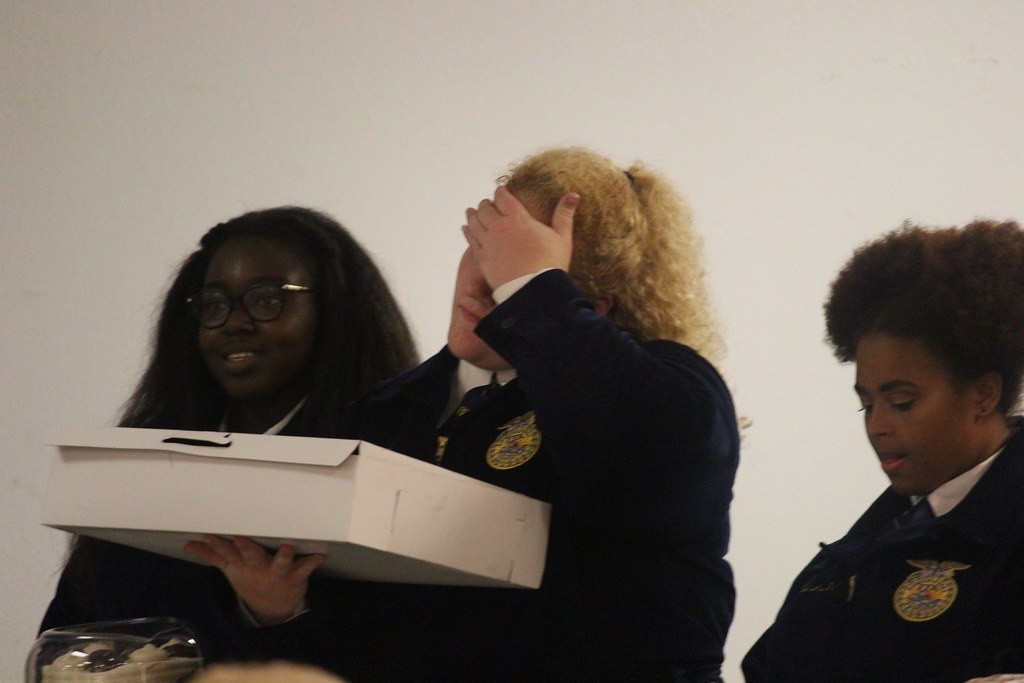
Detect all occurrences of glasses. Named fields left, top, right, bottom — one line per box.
left=188, top=284, right=350, bottom=330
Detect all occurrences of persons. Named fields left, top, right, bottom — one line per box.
left=33, top=207, right=420, bottom=683
left=739, top=222, right=1024, bottom=683
left=182, top=143, right=740, bottom=683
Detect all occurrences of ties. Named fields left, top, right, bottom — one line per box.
left=874, top=497, right=937, bottom=543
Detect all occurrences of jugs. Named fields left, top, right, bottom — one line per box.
left=26, top=617, right=203, bottom=683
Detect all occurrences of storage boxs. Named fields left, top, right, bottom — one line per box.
left=39, top=420, right=551, bottom=590
